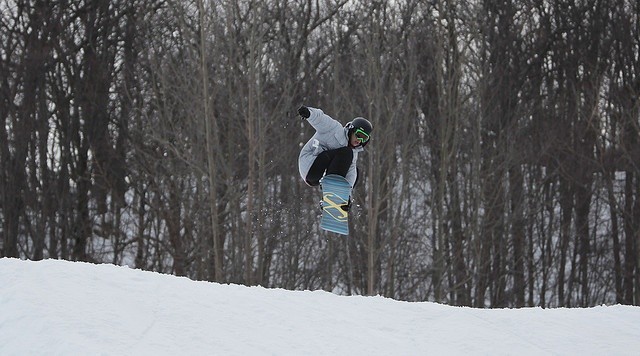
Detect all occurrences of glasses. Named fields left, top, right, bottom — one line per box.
left=355, top=129, right=370, bottom=143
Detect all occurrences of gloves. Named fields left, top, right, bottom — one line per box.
left=298, top=106, right=311, bottom=120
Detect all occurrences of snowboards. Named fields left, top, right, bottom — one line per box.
left=319, top=174, right=352, bottom=235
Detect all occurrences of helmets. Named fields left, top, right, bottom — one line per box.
left=347, top=117, right=372, bottom=147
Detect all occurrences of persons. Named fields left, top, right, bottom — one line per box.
left=298, top=106, right=373, bottom=208
